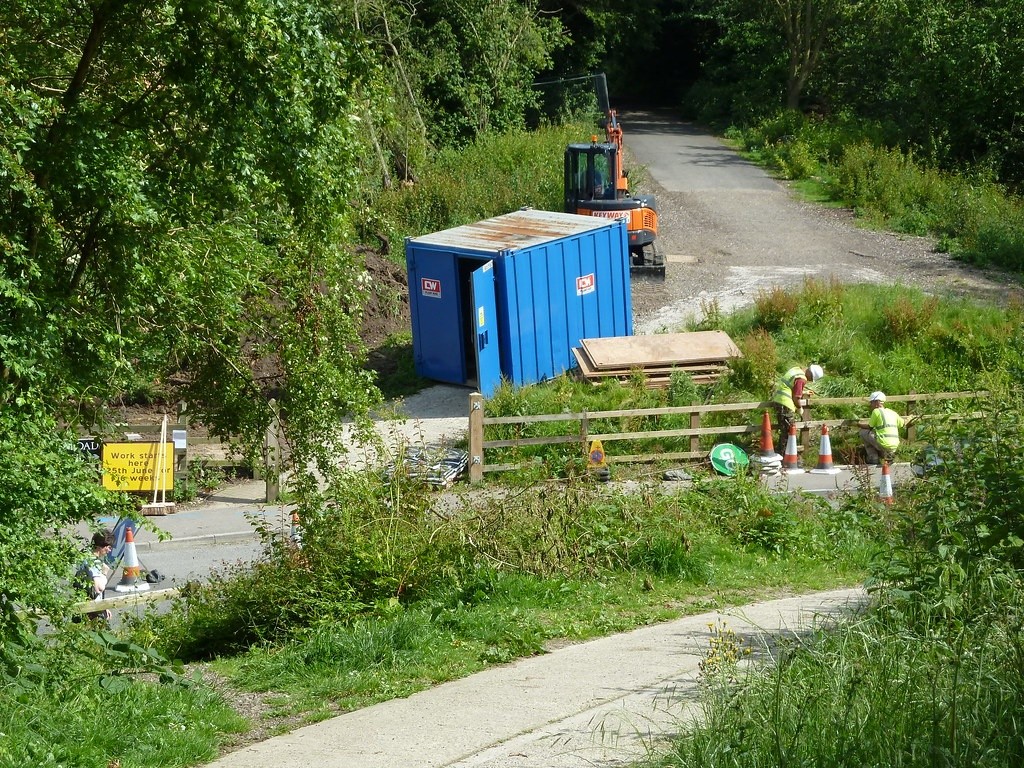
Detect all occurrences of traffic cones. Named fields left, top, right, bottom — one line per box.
left=117, top=526, right=144, bottom=585
left=754, top=410, right=777, bottom=457
left=814, top=423, right=836, bottom=469
left=286, top=512, right=309, bottom=566
left=875, top=461, right=895, bottom=505
left=781, top=424, right=802, bottom=469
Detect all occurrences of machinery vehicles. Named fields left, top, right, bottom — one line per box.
left=532, top=72, right=666, bottom=278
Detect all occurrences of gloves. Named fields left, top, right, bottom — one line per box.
left=802, top=390, right=814, bottom=396
left=797, top=406, right=804, bottom=415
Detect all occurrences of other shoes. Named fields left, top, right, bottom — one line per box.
left=866, top=457, right=878, bottom=463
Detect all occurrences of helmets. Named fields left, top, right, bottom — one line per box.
left=868, top=391, right=886, bottom=404
left=809, top=364, right=822, bottom=383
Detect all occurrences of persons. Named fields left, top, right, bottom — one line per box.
left=857, top=391, right=904, bottom=466
left=72, top=529, right=116, bottom=624
left=772, top=364, right=824, bottom=457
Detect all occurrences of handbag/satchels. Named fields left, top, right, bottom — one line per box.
left=72, top=569, right=97, bottom=599
